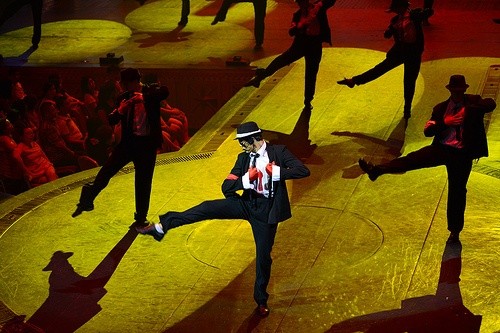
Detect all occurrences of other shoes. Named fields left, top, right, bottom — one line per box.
left=451, top=231, right=460, bottom=241
left=305, top=101, right=313, bottom=109
left=359, top=158, right=377, bottom=181
left=137, top=218, right=150, bottom=226
left=85, top=181, right=98, bottom=190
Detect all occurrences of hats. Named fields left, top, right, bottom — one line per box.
left=120, top=69, right=143, bottom=84
left=234, top=121, right=264, bottom=140
left=384, top=0, right=410, bottom=13
left=445, top=75, right=468, bottom=90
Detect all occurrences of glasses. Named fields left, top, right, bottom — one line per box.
left=241, top=140, right=250, bottom=148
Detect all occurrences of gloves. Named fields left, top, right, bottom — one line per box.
left=249, top=167, right=258, bottom=182
left=265, top=160, right=276, bottom=176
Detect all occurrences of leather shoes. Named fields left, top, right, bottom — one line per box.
left=259, top=304, right=270, bottom=315
left=135, top=222, right=163, bottom=242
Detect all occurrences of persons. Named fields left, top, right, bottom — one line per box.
left=72, top=68, right=170, bottom=227
left=0, top=0, right=43, bottom=49
left=211, top=0, right=267, bottom=53
left=132, top=121, right=310, bottom=317
left=359, top=75, right=497, bottom=251
left=240, top=0, right=337, bottom=110
left=137, top=0, right=190, bottom=26
left=336, top=0, right=434, bottom=117
left=0, top=54, right=191, bottom=195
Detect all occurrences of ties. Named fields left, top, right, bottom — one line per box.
left=451, top=107, right=460, bottom=141
left=251, top=154, right=260, bottom=190
left=128, top=100, right=137, bottom=129
